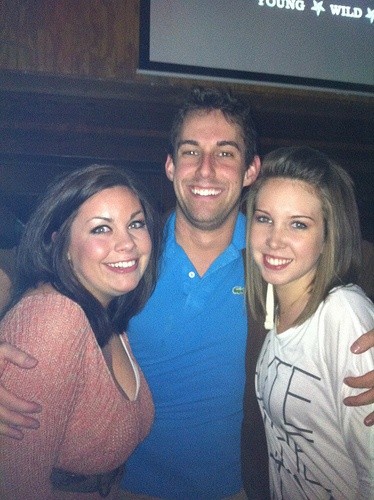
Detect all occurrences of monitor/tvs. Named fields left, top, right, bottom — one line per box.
left=137, top=0, right=374, bottom=98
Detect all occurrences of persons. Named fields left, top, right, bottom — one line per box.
left=244, top=145, right=374, bottom=500
left=0, top=88, right=374, bottom=500
left=0, top=163, right=154, bottom=500
